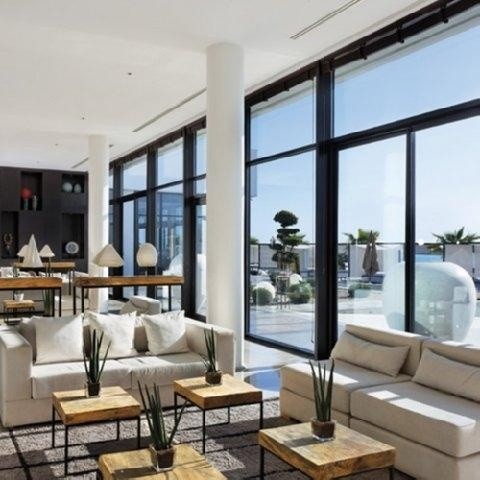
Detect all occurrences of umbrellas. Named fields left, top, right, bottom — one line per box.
left=360, top=230, right=380, bottom=287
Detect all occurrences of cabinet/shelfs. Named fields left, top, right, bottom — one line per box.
left=0, top=168, right=91, bottom=259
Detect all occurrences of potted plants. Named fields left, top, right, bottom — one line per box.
left=132, top=378, right=191, bottom=471
left=82, top=329, right=114, bottom=398
left=305, top=357, right=345, bottom=441
left=197, top=326, right=224, bottom=385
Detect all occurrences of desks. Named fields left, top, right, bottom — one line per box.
left=258, top=416, right=396, bottom=479
left=170, top=374, right=264, bottom=453
left=0, top=233, right=184, bottom=322
left=45, top=385, right=142, bottom=475
left=96, top=443, right=229, bottom=478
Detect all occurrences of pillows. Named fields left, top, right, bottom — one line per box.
left=412, top=347, right=480, bottom=402
left=330, top=328, right=411, bottom=378
left=140, top=310, right=187, bottom=356
left=35, top=314, right=86, bottom=365
left=85, top=311, right=141, bottom=361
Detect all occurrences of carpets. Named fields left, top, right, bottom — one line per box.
left=1, top=392, right=413, bottom=480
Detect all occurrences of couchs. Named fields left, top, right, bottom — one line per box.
left=276, top=320, right=480, bottom=480
left=0, top=310, right=235, bottom=430
